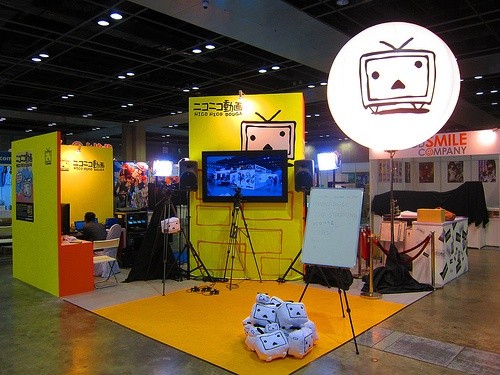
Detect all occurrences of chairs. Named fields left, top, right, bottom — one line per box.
left=92, top=224, right=122, bottom=289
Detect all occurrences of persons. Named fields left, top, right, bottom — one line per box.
left=448, top=161, right=456, bottom=182
left=483, top=160, right=497, bottom=182
left=83, top=212, right=107, bottom=251
left=266, top=176, right=278, bottom=192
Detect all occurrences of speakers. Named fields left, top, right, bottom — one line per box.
left=294, top=160, right=314, bottom=192
left=178, top=161, right=198, bottom=191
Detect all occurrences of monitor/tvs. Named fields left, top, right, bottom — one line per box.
left=74, top=221, right=86, bottom=231
left=202, top=150, right=288, bottom=203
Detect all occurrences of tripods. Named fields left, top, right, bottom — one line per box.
left=144, top=190, right=212, bottom=297
left=223, top=199, right=262, bottom=290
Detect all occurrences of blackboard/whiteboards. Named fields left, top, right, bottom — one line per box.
left=301, top=187, right=364, bottom=269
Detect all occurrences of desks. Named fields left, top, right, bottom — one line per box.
left=58, top=228, right=127, bottom=298
left=383, top=216, right=468, bottom=285
